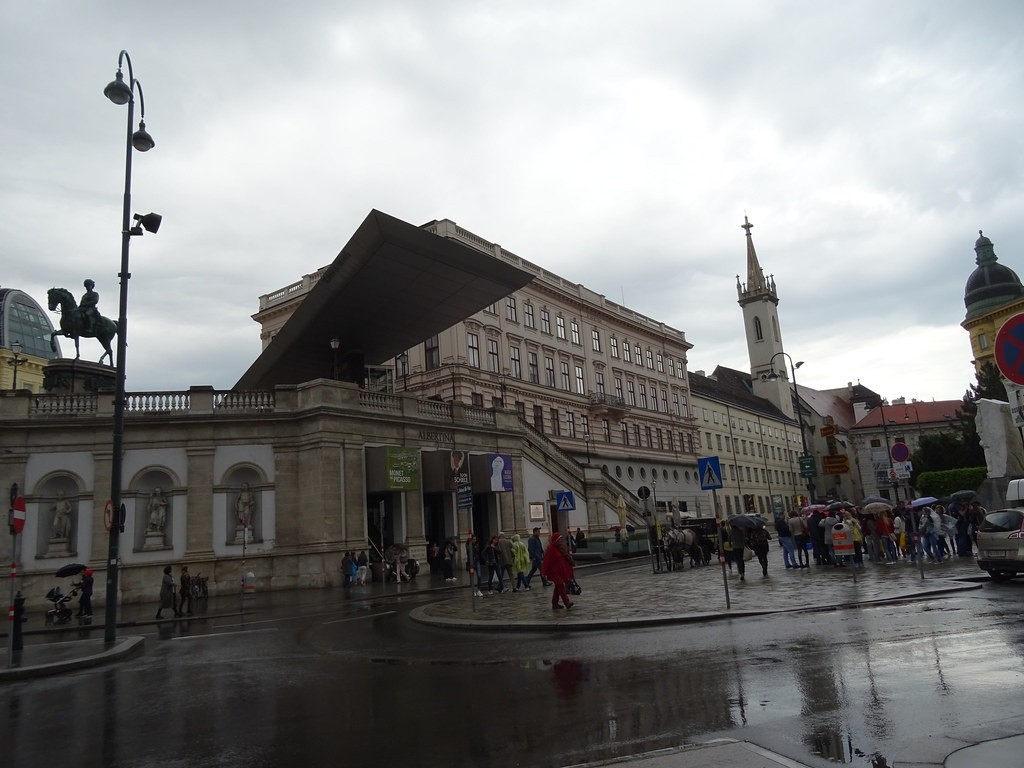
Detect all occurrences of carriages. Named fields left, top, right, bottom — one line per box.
left=661, top=516, right=720, bottom=571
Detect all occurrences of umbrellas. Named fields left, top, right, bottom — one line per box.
left=56, top=563, right=88, bottom=583
left=672, top=496, right=681, bottom=527
left=437, top=536, right=460, bottom=547
left=696, top=496, right=701, bottom=519
left=616, top=495, right=628, bottom=543
left=388, top=544, right=408, bottom=551
left=609, top=525, right=621, bottom=530
left=725, top=490, right=978, bottom=531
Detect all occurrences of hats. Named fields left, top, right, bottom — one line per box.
left=533, top=527, right=541, bottom=531
left=84, top=569, right=93, bottom=576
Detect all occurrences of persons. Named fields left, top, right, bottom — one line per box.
left=56, top=587, right=65, bottom=608
left=74, top=576, right=89, bottom=616
left=179, top=566, right=193, bottom=614
left=431, top=542, right=457, bottom=581
left=465, top=527, right=584, bottom=609
left=395, top=548, right=410, bottom=583
left=81, top=570, right=94, bottom=615
left=720, top=501, right=987, bottom=579
left=615, top=529, right=620, bottom=542
left=342, top=551, right=368, bottom=586
left=234, top=482, right=254, bottom=527
left=50, top=491, right=71, bottom=538
left=145, top=487, right=168, bottom=532
left=156, top=566, right=182, bottom=619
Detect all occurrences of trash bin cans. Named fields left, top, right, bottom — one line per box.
left=404, top=558, right=416, bottom=578
left=831, top=523, right=856, bottom=555
left=186, top=578, right=211, bottom=613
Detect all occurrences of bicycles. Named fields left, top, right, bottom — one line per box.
left=191, top=573, right=209, bottom=600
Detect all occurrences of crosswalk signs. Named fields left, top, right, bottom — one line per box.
left=556, top=491, right=576, bottom=511
left=697, top=456, right=724, bottom=490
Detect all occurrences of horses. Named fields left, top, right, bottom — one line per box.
left=46, top=288, right=129, bottom=367
left=652, top=525, right=700, bottom=571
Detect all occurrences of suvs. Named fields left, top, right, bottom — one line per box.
left=977, top=508, right=1024, bottom=581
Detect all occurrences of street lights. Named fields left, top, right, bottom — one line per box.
left=104, top=49, right=154, bottom=641
left=7, top=339, right=28, bottom=389
left=904, top=405, right=922, bottom=436
left=651, top=479, right=658, bottom=509
left=864, top=396, right=899, bottom=503
left=766, top=352, right=816, bottom=504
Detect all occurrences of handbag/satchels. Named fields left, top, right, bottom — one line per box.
left=803, top=531, right=811, bottom=543
left=743, top=546, right=752, bottom=561
left=492, top=569, right=499, bottom=584
left=564, top=579, right=582, bottom=595
left=889, top=533, right=897, bottom=541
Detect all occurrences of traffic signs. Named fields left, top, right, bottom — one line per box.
left=823, top=456, right=849, bottom=474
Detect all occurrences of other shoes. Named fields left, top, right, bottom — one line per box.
left=408, top=576, right=412, bottom=582
left=800, top=564, right=805, bottom=569
left=175, top=613, right=182, bottom=617
left=763, top=567, right=767, bottom=576
left=75, top=612, right=93, bottom=619
left=565, top=602, right=574, bottom=609
left=186, top=611, right=194, bottom=615
left=156, top=615, right=165, bottom=619
left=805, top=563, right=809, bottom=567
left=817, top=551, right=974, bottom=568
left=740, top=574, right=745, bottom=580
left=786, top=564, right=793, bottom=568
left=472, top=582, right=552, bottom=596
left=552, top=604, right=564, bottom=609
left=793, top=564, right=799, bottom=569
left=446, top=577, right=457, bottom=580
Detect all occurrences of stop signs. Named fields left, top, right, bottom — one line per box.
left=13, top=495, right=26, bottom=533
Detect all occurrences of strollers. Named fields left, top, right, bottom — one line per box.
left=45, top=587, right=79, bottom=619
